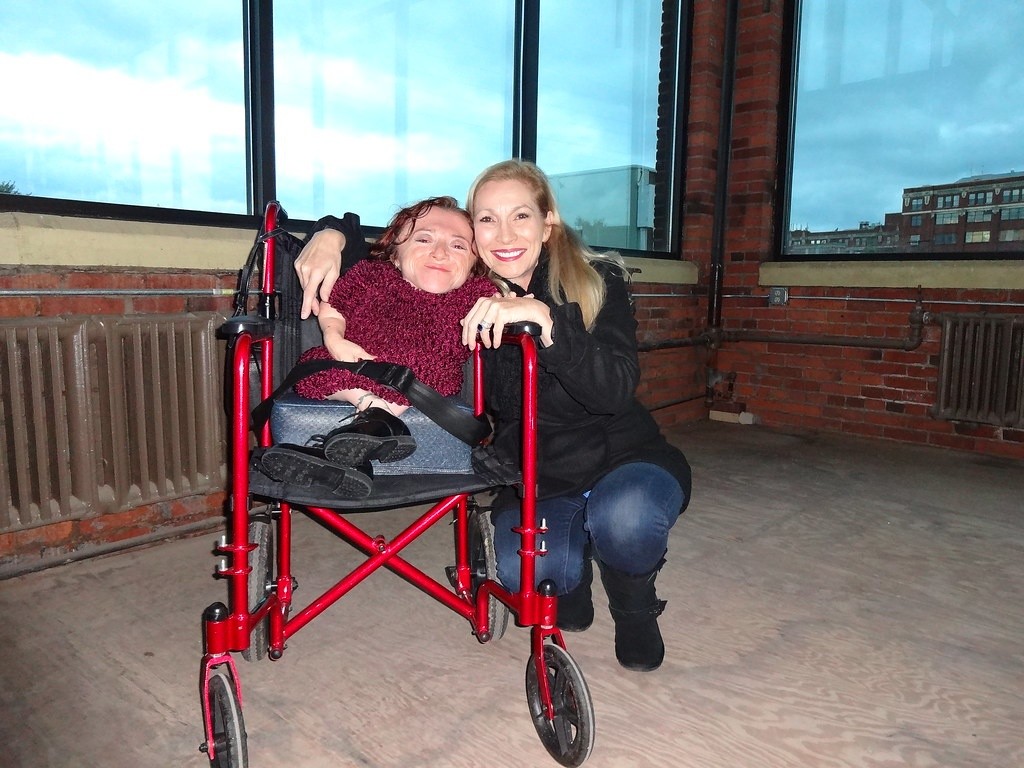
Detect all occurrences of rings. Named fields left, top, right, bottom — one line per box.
left=477, top=319, right=494, bottom=333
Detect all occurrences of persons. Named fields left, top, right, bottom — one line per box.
left=288, top=153, right=697, bottom=675
left=263, top=194, right=534, bottom=515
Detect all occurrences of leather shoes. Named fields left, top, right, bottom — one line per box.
left=261, top=441, right=375, bottom=499
left=323, top=406, right=418, bottom=467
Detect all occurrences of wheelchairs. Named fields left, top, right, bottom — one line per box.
left=194, top=201, right=596, bottom=768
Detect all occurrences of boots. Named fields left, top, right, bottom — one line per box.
left=595, top=548, right=669, bottom=671
left=555, top=543, right=594, bottom=631
